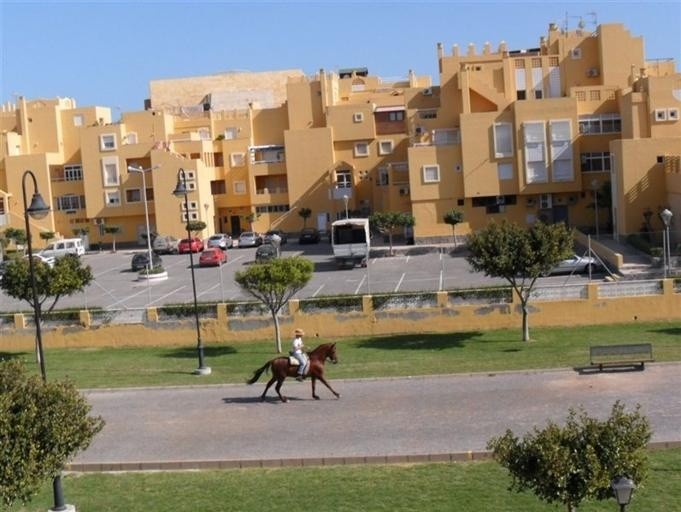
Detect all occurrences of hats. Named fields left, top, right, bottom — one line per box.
left=294, top=329, right=304, bottom=336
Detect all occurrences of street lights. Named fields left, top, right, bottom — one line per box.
left=171, top=165, right=210, bottom=374
left=16, top=167, right=84, bottom=510
left=660, top=206, right=674, bottom=278
left=340, top=193, right=350, bottom=220
left=591, top=178, right=601, bottom=242
left=124, top=161, right=161, bottom=273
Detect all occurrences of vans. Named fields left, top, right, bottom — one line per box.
left=39, top=238, right=85, bottom=261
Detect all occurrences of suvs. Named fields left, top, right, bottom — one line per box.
left=133, top=251, right=164, bottom=269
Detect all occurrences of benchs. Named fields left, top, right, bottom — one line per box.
left=590, top=344, right=655, bottom=372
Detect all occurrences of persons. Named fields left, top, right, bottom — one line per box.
left=292, top=328, right=307, bottom=382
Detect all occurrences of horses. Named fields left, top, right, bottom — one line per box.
left=245, top=341, right=342, bottom=403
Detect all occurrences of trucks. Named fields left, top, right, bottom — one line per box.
left=329, top=217, right=373, bottom=269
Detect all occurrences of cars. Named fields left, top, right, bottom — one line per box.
left=254, top=244, right=281, bottom=261
left=151, top=228, right=288, bottom=256
left=298, top=227, right=323, bottom=245
left=20, top=253, right=56, bottom=270
left=512, top=251, right=603, bottom=278
left=197, top=247, right=229, bottom=267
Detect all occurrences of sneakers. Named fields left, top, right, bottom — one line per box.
left=296, top=376, right=303, bottom=381
